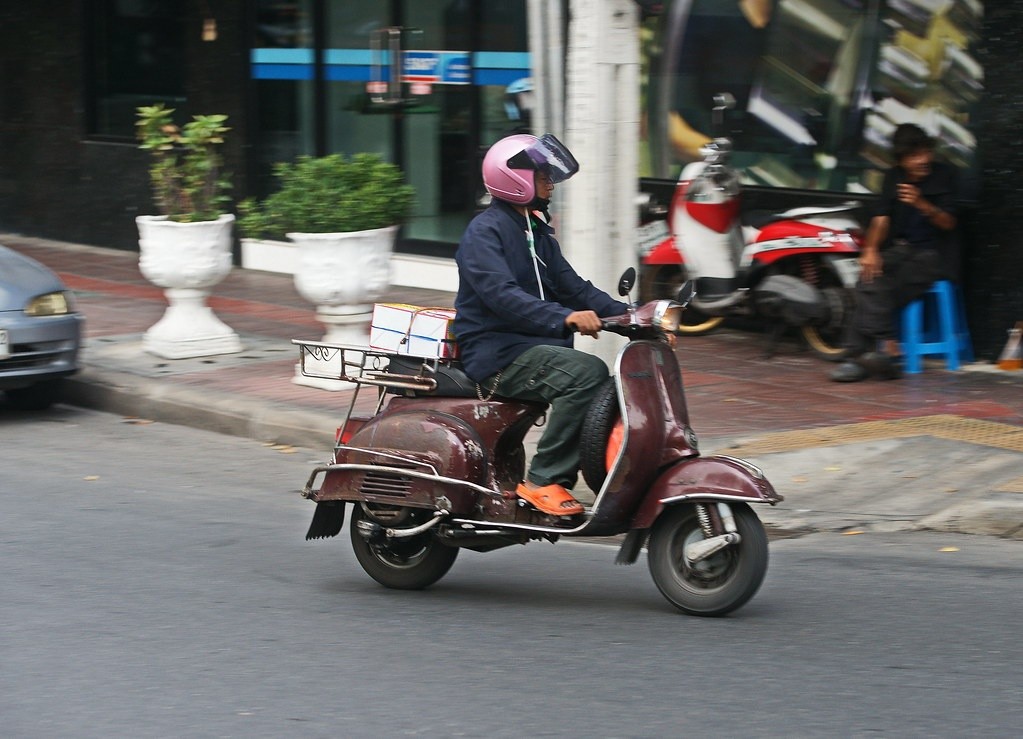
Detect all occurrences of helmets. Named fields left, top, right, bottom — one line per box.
left=481, top=134, right=553, bottom=207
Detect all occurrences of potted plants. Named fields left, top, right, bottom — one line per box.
left=131, top=103, right=238, bottom=362
left=237, top=151, right=419, bottom=392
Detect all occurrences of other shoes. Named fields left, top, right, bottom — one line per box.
left=821, top=352, right=891, bottom=383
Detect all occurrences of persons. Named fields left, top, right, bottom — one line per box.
left=449, top=133, right=675, bottom=515
left=825, top=122, right=965, bottom=382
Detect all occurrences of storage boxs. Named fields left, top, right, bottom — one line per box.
left=370, top=302, right=462, bottom=364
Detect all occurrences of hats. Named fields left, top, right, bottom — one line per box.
left=892, top=123, right=937, bottom=156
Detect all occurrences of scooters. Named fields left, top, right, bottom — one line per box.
left=636, top=125, right=872, bottom=363
left=290, top=266, right=787, bottom=618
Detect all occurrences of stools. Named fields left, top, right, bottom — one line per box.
left=897, top=281, right=971, bottom=376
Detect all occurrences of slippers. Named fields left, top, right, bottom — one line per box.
left=515, top=483, right=584, bottom=516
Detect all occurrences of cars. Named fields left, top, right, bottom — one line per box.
left=0, top=244, right=87, bottom=413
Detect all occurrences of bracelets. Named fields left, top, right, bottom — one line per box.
left=921, top=208, right=941, bottom=218
